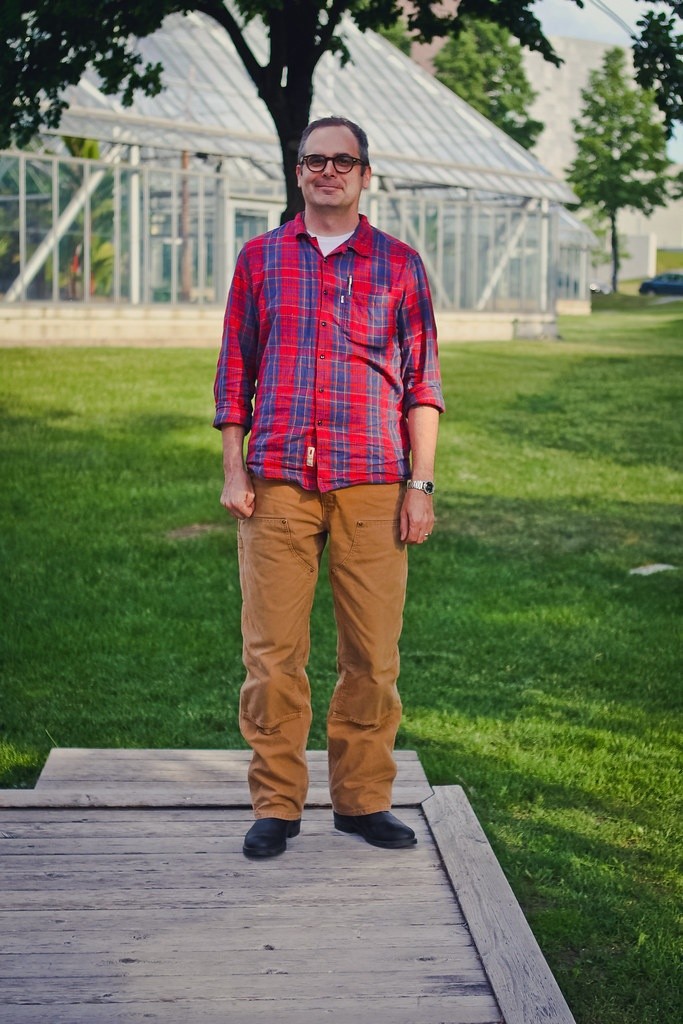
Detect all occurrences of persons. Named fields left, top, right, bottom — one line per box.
left=212, top=116, right=445, bottom=861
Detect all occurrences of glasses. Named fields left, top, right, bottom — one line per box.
left=300, top=154, right=365, bottom=174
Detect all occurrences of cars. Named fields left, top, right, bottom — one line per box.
left=639, top=272, right=683, bottom=297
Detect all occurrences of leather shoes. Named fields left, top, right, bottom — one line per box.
left=330, top=812, right=420, bottom=849
left=239, top=820, right=301, bottom=860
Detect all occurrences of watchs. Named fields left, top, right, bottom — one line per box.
left=407, top=479, right=436, bottom=496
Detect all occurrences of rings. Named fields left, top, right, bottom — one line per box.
left=424, top=533, right=429, bottom=539
